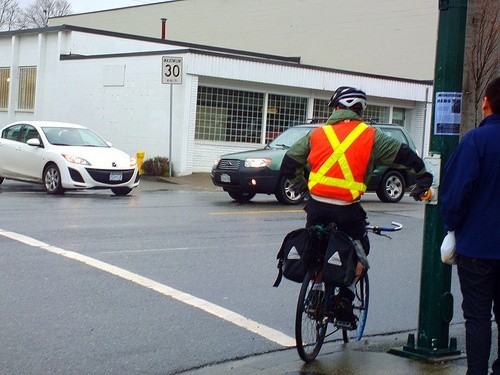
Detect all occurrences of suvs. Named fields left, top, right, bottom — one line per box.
left=210, top=117, right=421, bottom=206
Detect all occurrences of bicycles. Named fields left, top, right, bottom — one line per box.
left=295, top=221, right=404, bottom=363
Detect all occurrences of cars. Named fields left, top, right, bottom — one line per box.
left=0, top=120, right=141, bottom=197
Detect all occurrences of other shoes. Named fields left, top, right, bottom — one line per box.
left=323, top=295, right=358, bottom=331
left=306, top=290, right=325, bottom=321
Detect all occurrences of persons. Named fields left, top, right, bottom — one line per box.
left=439, top=78, right=500, bottom=375
left=279, top=86, right=433, bottom=323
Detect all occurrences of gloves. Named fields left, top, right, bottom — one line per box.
left=409, top=186, right=435, bottom=201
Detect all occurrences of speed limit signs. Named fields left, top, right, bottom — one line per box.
left=161, top=55, right=184, bottom=84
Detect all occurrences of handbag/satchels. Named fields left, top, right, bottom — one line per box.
left=320, top=232, right=358, bottom=288
left=276, top=227, right=321, bottom=284
left=440, top=230, right=458, bottom=265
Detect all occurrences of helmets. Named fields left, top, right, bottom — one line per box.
left=327, top=86, right=367, bottom=116
